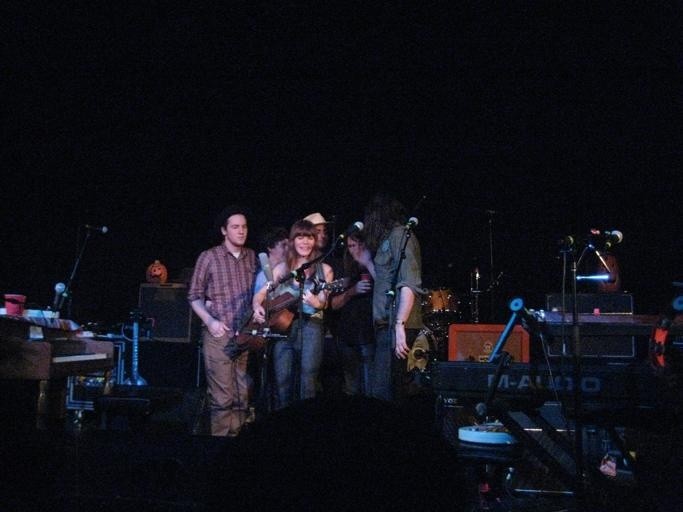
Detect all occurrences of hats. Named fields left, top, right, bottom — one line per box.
left=302, top=212, right=327, bottom=225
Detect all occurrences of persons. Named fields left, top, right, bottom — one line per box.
left=187, top=206, right=255, bottom=437
left=253, top=189, right=423, bottom=438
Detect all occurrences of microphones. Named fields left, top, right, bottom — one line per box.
left=256, top=244, right=274, bottom=281
left=402, top=217, right=419, bottom=232
left=476, top=362, right=506, bottom=416
left=608, top=230, right=625, bottom=250
left=577, top=272, right=617, bottom=284
left=384, top=290, right=395, bottom=310
left=413, top=349, right=423, bottom=360
left=84, top=222, right=107, bottom=235
left=595, top=251, right=611, bottom=273
left=554, top=235, right=574, bottom=248
left=339, top=220, right=364, bottom=240
left=52, top=282, right=66, bottom=308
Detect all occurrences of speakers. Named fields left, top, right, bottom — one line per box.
left=126, top=342, right=200, bottom=389
left=545, top=293, right=638, bottom=359
left=135, top=283, right=191, bottom=343
left=448, top=324, right=529, bottom=363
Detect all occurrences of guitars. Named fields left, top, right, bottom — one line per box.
left=264, top=278, right=344, bottom=333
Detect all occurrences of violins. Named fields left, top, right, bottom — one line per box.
left=223, top=322, right=288, bottom=360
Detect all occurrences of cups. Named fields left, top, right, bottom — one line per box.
left=4, top=294, right=26, bottom=317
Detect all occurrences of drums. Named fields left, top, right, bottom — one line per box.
left=407, top=329, right=439, bottom=384
left=422, top=287, right=464, bottom=336
